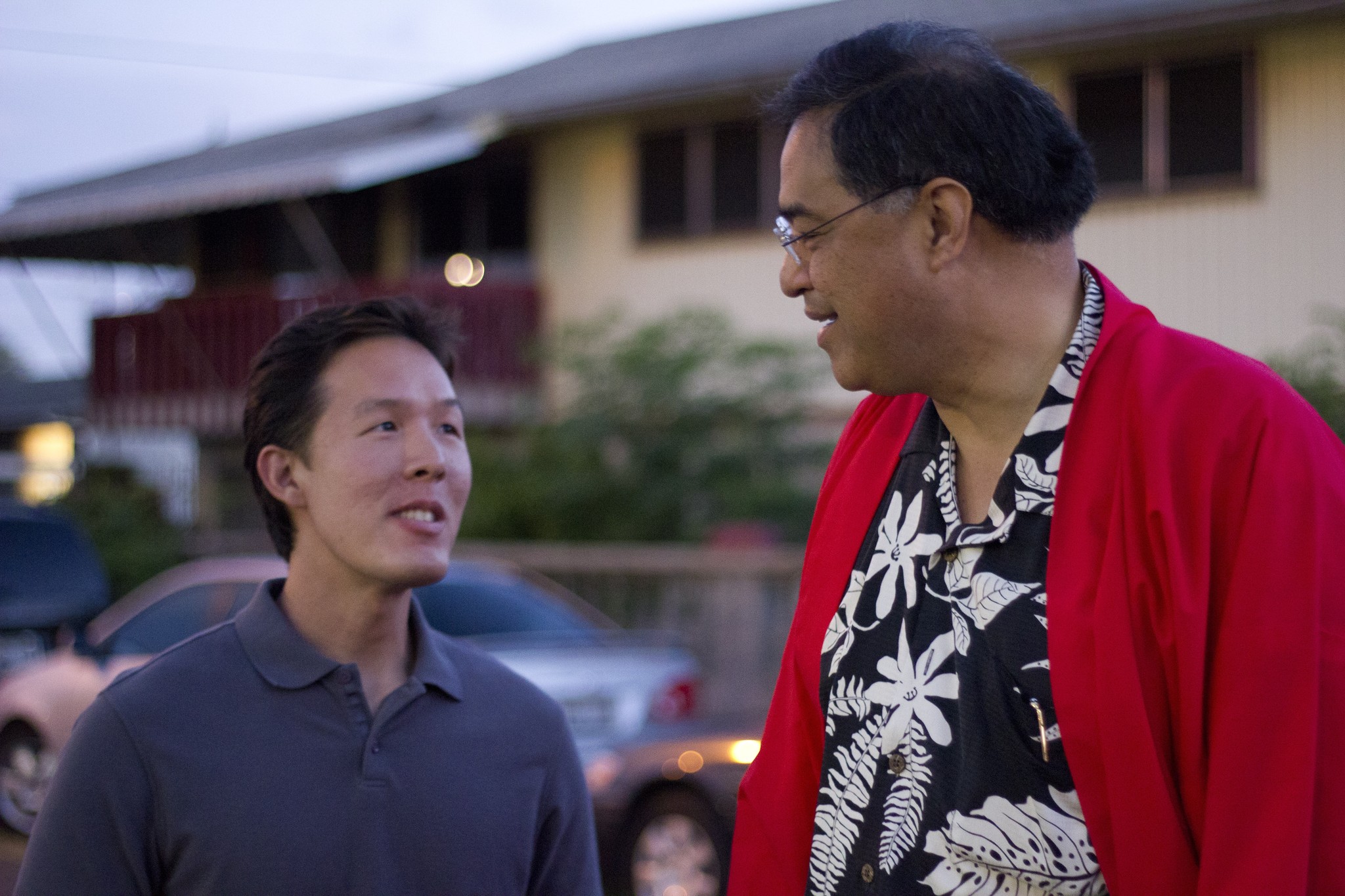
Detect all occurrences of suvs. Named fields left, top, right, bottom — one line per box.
left=0, top=502, right=117, bottom=687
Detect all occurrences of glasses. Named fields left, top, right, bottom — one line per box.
left=773, top=181, right=930, bottom=266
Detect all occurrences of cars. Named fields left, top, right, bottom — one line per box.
left=1, top=551, right=694, bottom=840
left=579, top=717, right=759, bottom=896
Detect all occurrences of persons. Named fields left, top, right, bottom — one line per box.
left=17, top=300, right=610, bottom=896
left=720, top=5, right=1345, bottom=896
